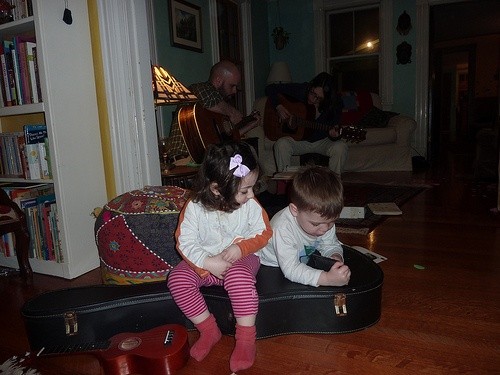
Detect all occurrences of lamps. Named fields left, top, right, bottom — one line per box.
left=150, top=64, right=197, bottom=173
left=269, top=64, right=291, bottom=85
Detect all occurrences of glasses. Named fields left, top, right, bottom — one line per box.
left=310, top=90, right=320, bottom=103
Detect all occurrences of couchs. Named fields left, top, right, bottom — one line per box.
left=254, top=90, right=417, bottom=176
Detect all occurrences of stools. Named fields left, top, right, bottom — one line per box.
left=95, top=184, right=197, bottom=285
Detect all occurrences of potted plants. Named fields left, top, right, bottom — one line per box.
left=272, top=25, right=290, bottom=49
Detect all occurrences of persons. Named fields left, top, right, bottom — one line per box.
left=266, top=72, right=348, bottom=178
left=261, top=167, right=351, bottom=287
left=167, top=140, right=273, bottom=371
left=164, top=60, right=273, bottom=210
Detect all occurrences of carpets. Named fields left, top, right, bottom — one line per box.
left=253, top=180, right=427, bottom=234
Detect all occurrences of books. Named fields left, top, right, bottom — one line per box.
left=0, top=125, right=52, bottom=179
left=368, top=203, right=402, bottom=215
left=0, top=183, right=64, bottom=264
left=0, top=36, right=42, bottom=108
left=7, top=0, right=33, bottom=21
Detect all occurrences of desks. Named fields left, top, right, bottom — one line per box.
left=162, top=165, right=202, bottom=194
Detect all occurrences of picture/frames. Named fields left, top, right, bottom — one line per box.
left=168, top=0, right=204, bottom=53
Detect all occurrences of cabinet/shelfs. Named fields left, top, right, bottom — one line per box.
left=0, top=0, right=111, bottom=281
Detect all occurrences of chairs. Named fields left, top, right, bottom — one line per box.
left=0, top=188, right=34, bottom=284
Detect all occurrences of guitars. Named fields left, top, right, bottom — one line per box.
left=34, top=324, right=191, bottom=375
left=264, top=103, right=368, bottom=144
left=178, top=102, right=260, bottom=164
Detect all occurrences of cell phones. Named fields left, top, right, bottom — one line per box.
left=307, top=255, right=336, bottom=272
